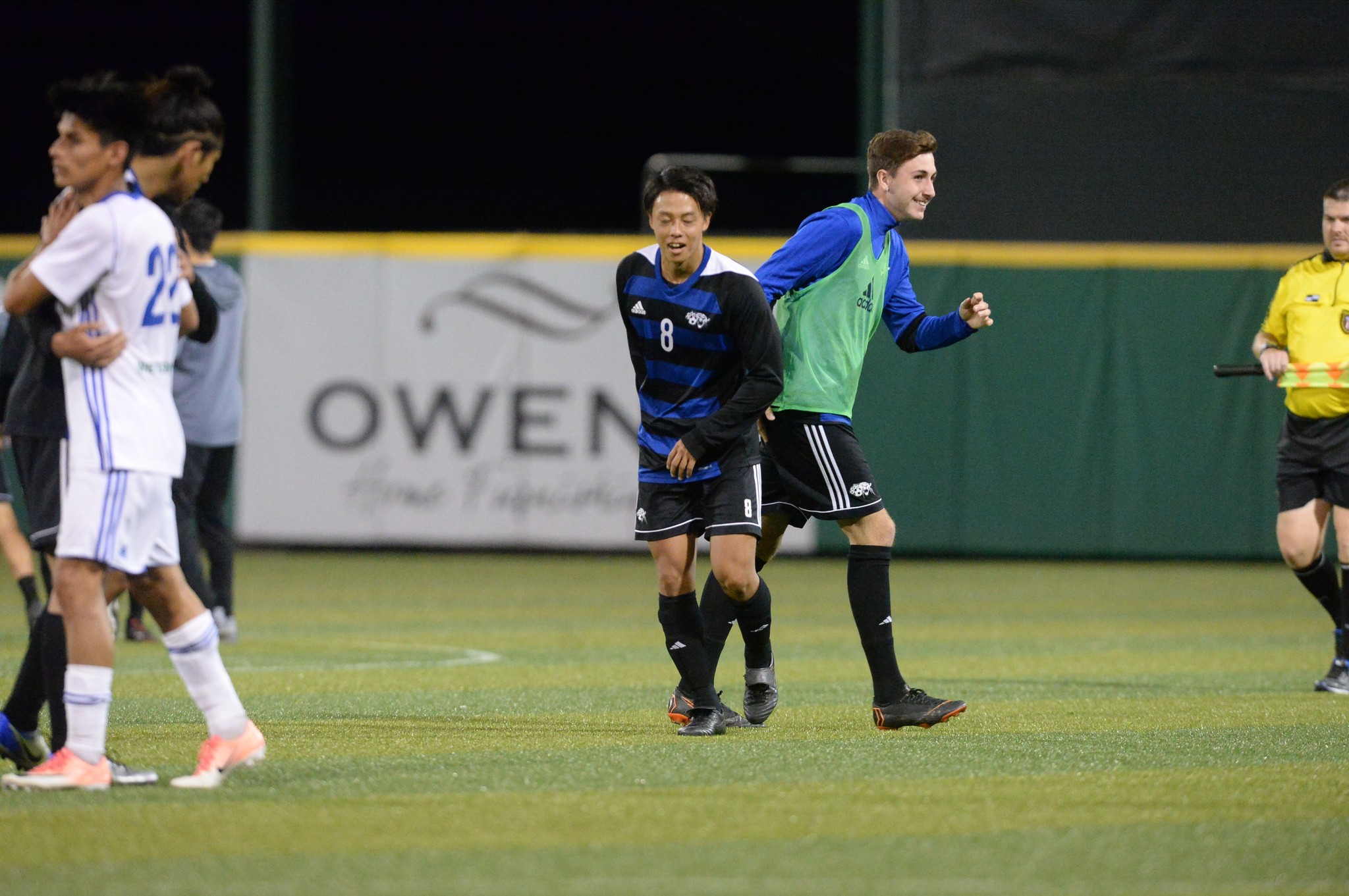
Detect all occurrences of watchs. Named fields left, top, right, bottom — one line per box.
left=1257, top=342, right=1283, bottom=356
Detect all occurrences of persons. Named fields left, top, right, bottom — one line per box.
left=657, top=126, right=999, bottom=731
left=0, top=426, right=48, bottom=650
left=119, top=587, right=158, bottom=648
left=1248, top=175, right=1349, bottom=700
left=158, top=198, right=253, bottom=645
left=0, top=84, right=234, bottom=775
left=1, top=91, right=270, bottom=799
left=610, top=162, right=783, bottom=745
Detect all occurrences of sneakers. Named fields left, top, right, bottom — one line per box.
left=668, top=687, right=764, bottom=728
left=872, top=685, right=966, bottom=729
left=125, top=618, right=152, bottom=642
left=675, top=708, right=727, bottom=736
left=110, top=757, right=158, bottom=784
left=1313, top=626, right=1349, bottom=693
left=1, top=708, right=52, bottom=769
left=170, top=719, right=265, bottom=788
left=3, top=746, right=111, bottom=792
left=742, top=644, right=777, bottom=723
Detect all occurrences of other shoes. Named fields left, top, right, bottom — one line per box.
left=213, top=606, right=237, bottom=640
